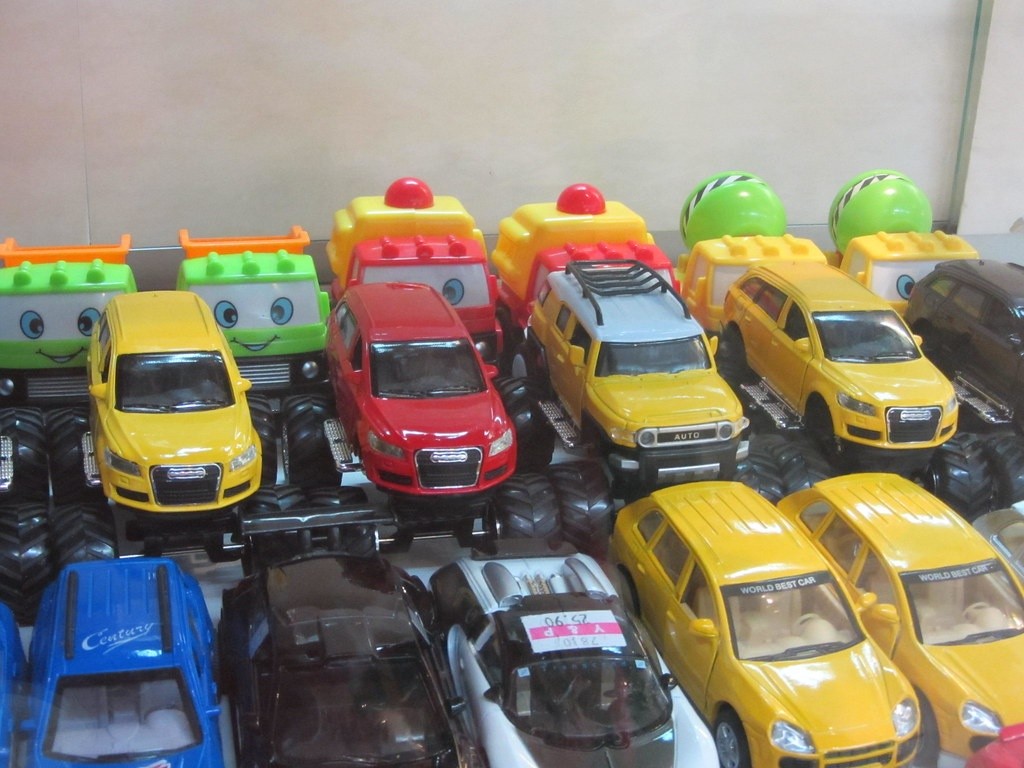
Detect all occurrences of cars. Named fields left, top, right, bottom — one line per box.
left=772, top=469, right=1024, bottom=761
left=0, top=595, right=32, bottom=768
left=16, top=554, right=223, bottom=768
left=218, top=509, right=468, bottom=768
left=426, top=549, right=721, bottom=768
left=607, top=479, right=922, bottom=768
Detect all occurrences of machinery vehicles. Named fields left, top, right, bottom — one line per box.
left=827, top=167, right=983, bottom=335
left=172, top=226, right=330, bottom=394
left=672, top=170, right=828, bottom=335
left=0, top=231, right=142, bottom=404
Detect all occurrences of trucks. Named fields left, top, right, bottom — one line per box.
left=325, top=176, right=501, bottom=361
left=491, top=180, right=679, bottom=345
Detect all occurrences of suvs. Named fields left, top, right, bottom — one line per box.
left=510, top=257, right=752, bottom=487
left=903, top=254, right=1023, bottom=424
left=320, top=282, right=518, bottom=493
left=716, top=263, right=958, bottom=459
left=77, top=290, right=262, bottom=522
